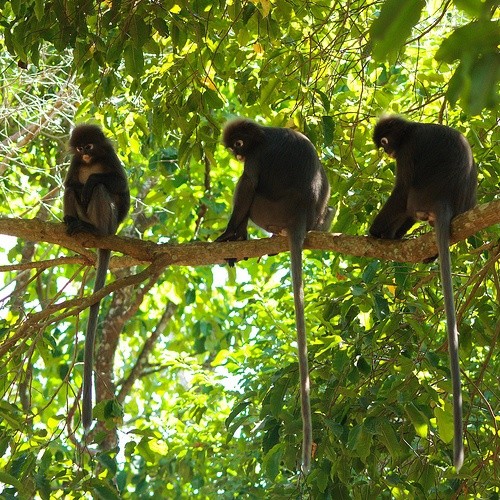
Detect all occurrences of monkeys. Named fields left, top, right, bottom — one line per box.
left=212, top=119, right=330, bottom=473
left=61, top=126, right=130, bottom=430
left=370, top=117, right=478, bottom=473
